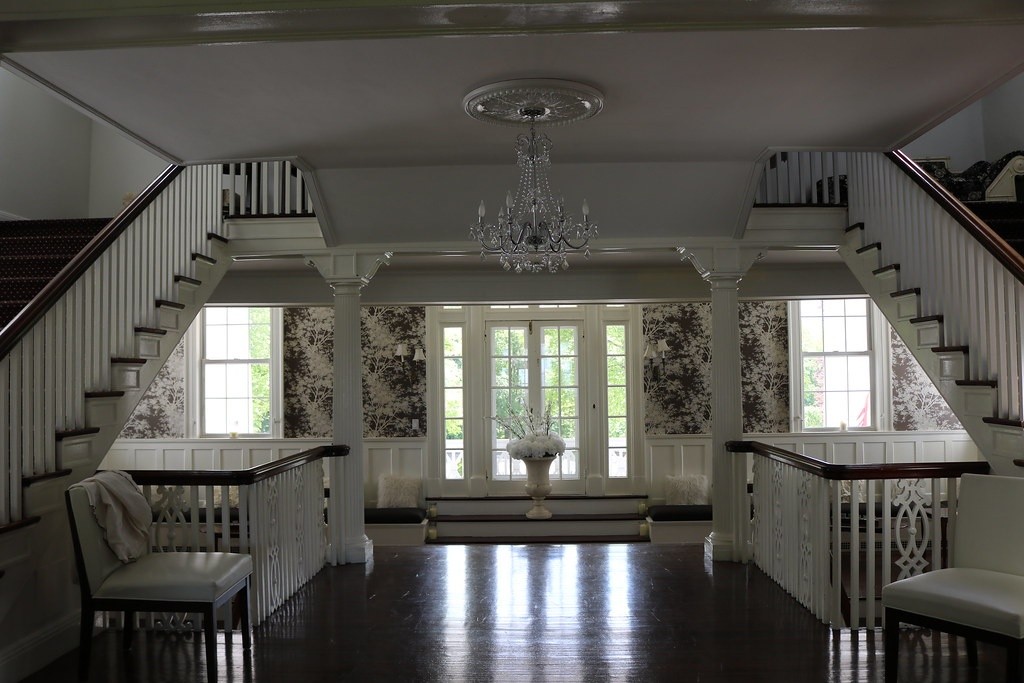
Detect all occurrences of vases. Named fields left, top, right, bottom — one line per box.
left=520, top=456, right=557, bottom=519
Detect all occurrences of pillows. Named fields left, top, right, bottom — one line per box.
left=950, top=173, right=982, bottom=193
left=829, top=479, right=867, bottom=503
left=377, top=473, right=422, bottom=508
left=203, top=485, right=240, bottom=508
left=966, top=161, right=992, bottom=183
left=665, top=474, right=709, bottom=506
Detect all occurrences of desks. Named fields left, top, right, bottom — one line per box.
left=910, top=154, right=952, bottom=173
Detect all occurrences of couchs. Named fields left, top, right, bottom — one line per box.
left=810, top=150, right=1024, bottom=204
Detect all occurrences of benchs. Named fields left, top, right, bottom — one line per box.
left=645, top=499, right=959, bottom=546
left=147, top=505, right=430, bottom=547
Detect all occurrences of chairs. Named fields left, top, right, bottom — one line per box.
left=63, top=468, right=253, bottom=683
left=880, top=473, right=1024, bottom=683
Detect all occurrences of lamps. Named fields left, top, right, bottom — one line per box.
left=461, top=77, right=606, bottom=275
left=640, top=338, right=672, bottom=401
left=395, top=343, right=424, bottom=395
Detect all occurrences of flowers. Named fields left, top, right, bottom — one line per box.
left=480, top=399, right=566, bottom=460
